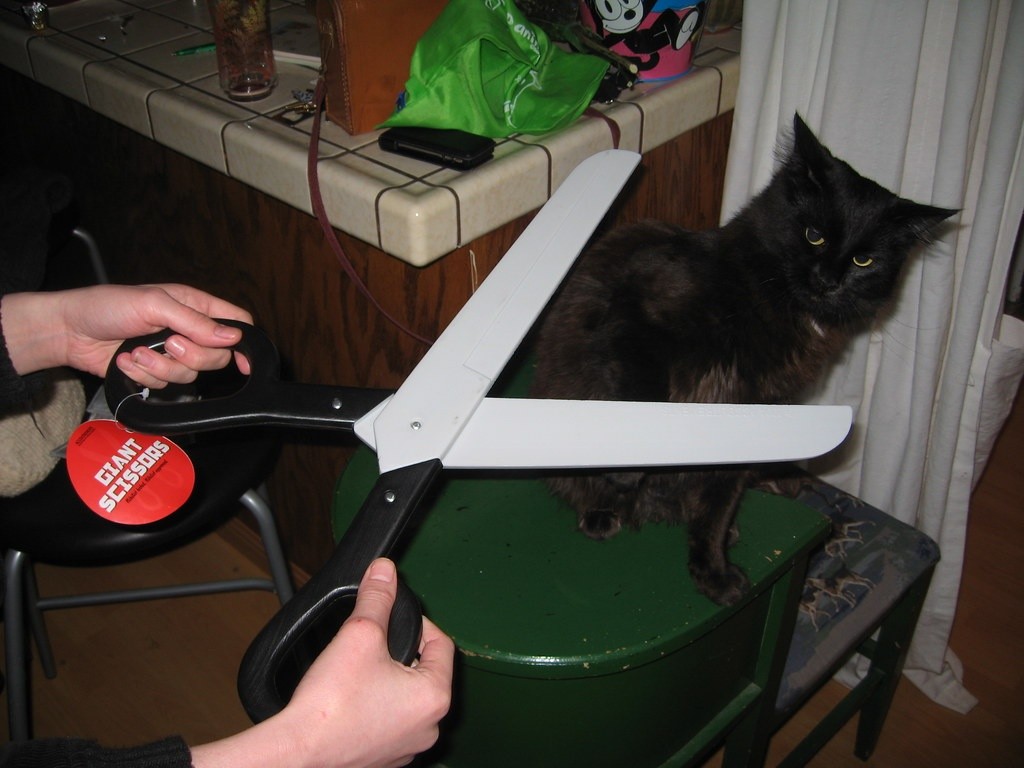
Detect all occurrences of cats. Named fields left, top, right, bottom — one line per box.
left=523, top=112, right=962, bottom=610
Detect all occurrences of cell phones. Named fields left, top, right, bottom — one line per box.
left=378, top=127, right=496, bottom=173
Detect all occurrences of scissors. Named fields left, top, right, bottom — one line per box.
left=101, top=148, right=857, bottom=725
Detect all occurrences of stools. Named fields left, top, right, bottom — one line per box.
left=0, top=189, right=117, bottom=294
left=721, top=462, right=944, bottom=768
left=0, top=356, right=299, bottom=742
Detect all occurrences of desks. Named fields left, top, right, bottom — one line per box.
left=0, top=0, right=744, bottom=579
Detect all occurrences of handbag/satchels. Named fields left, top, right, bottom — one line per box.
left=317, top=0, right=450, bottom=135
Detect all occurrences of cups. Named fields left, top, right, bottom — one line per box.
left=580, top=0, right=708, bottom=82
left=21, top=3, right=50, bottom=32
left=206, top=0, right=277, bottom=102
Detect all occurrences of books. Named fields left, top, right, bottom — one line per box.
left=270, top=19, right=324, bottom=67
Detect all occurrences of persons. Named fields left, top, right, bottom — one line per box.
left=0, top=283, right=457, bottom=768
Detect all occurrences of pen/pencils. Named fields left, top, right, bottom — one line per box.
left=171, top=44, right=216, bottom=58
